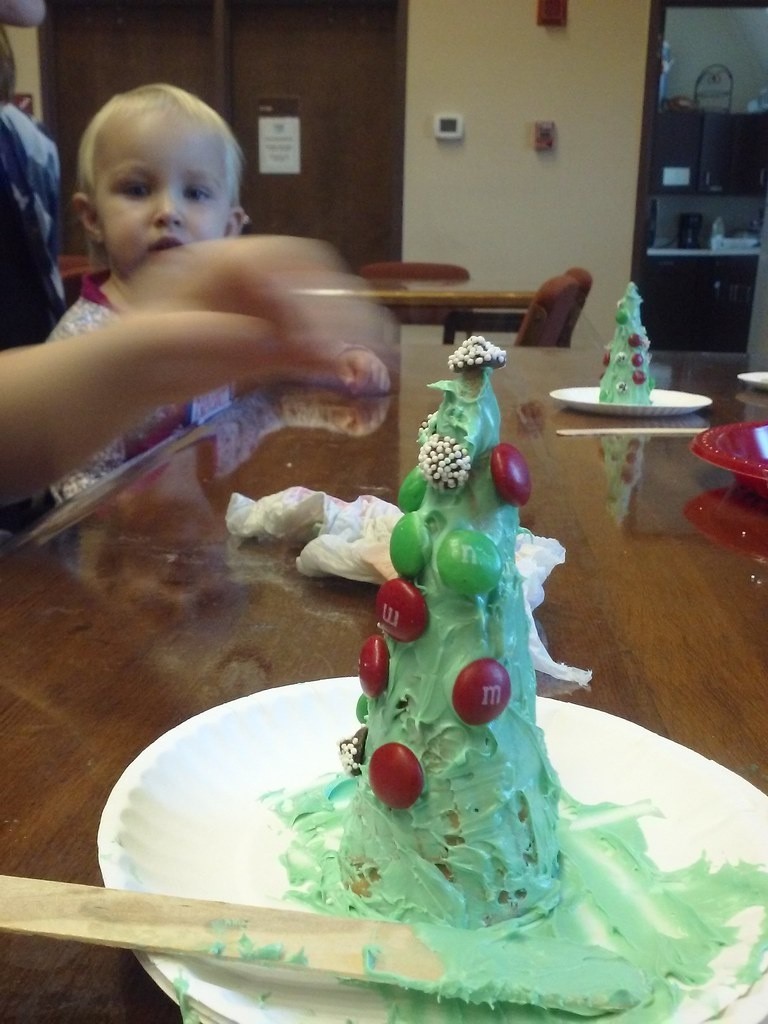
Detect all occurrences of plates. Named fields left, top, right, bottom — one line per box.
left=545, top=384, right=714, bottom=417
left=94, top=667, right=768, bottom=1021
left=736, top=371, right=767, bottom=391
left=688, top=418, right=768, bottom=499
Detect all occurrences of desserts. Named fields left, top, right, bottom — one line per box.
left=599, top=281, right=658, bottom=406
left=286, top=334, right=570, bottom=926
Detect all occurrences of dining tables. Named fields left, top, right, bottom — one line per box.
left=1, top=340, right=766, bottom=1024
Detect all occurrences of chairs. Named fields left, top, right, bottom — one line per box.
left=358, top=260, right=473, bottom=329
left=514, top=269, right=595, bottom=353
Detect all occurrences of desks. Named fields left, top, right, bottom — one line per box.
left=334, top=264, right=541, bottom=331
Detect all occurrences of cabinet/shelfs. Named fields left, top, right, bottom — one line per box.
left=637, top=249, right=760, bottom=352
left=650, top=106, right=766, bottom=200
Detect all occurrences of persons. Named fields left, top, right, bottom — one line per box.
left=2, top=1, right=394, bottom=547
left=40, top=83, right=389, bottom=511
